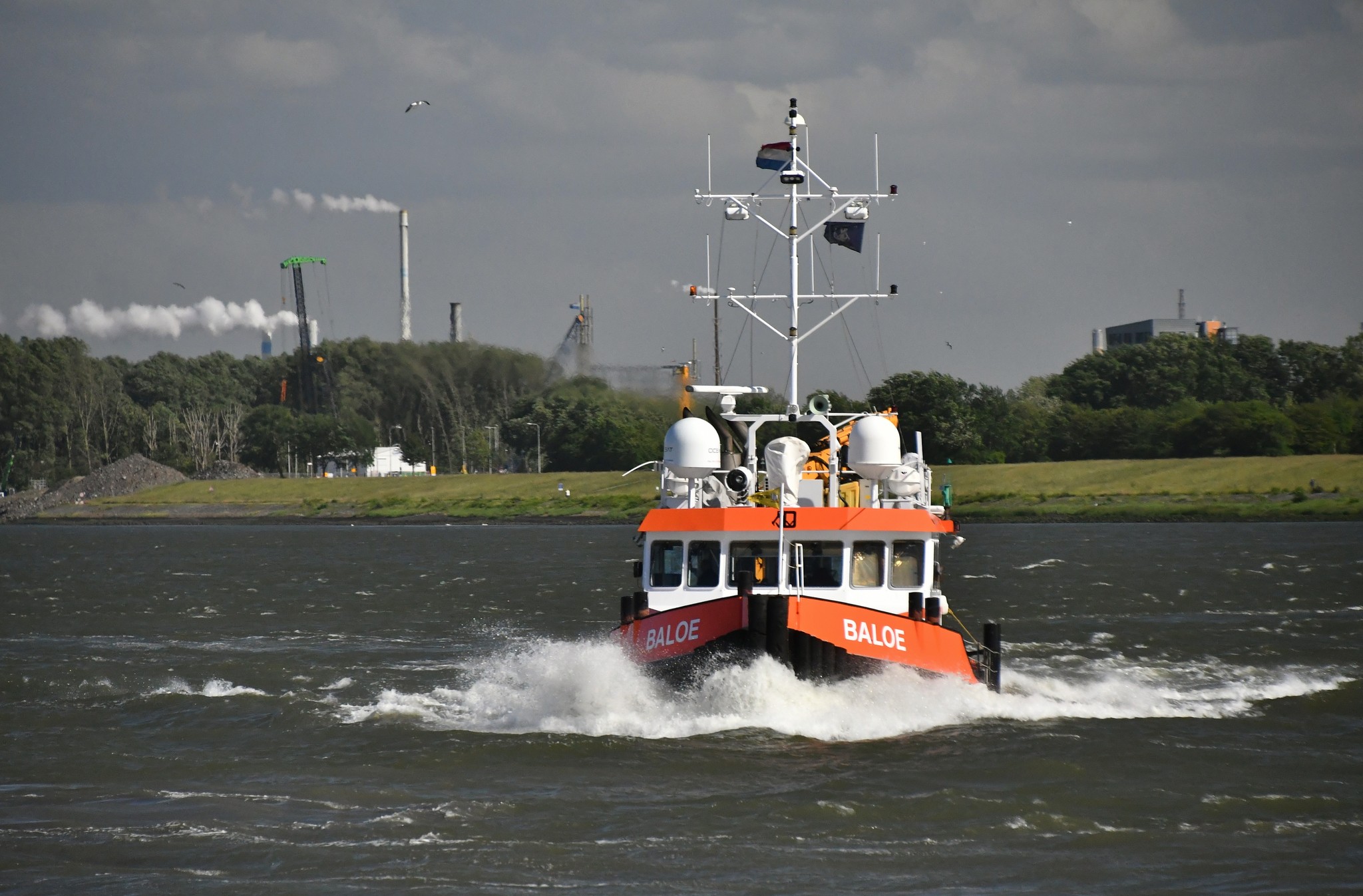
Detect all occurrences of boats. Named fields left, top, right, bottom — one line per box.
left=607, top=1, right=1008, bottom=701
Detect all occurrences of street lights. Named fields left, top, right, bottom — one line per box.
left=389, top=425, right=403, bottom=447
left=526, top=423, right=540, bottom=473
left=484, top=424, right=500, bottom=474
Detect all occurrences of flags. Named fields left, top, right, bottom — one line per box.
left=755, top=142, right=794, bottom=172
left=824, top=221, right=865, bottom=252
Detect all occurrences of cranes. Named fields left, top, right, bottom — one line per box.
left=280, top=256, right=327, bottom=404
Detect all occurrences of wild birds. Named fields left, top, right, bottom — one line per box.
left=405, top=100, right=430, bottom=113
left=172, top=282, right=185, bottom=290
